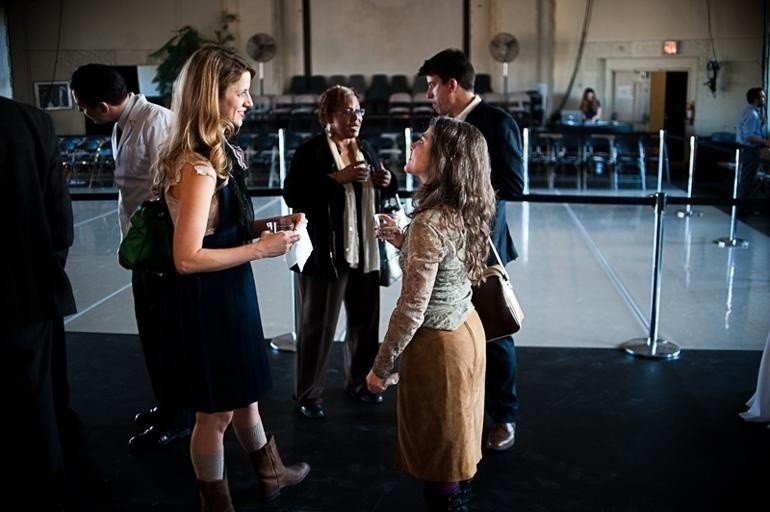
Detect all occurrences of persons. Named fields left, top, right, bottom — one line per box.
left=0, top=95, right=80, bottom=503
left=70, top=64, right=189, bottom=453
left=365, top=117, right=492, bottom=510
left=159, top=44, right=312, bottom=512
left=282, top=82, right=397, bottom=415
left=735, top=87, right=770, bottom=221
left=579, top=88, right=602, bottom=121
left=420, top=48, right=527, bottom=449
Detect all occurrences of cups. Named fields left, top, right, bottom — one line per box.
left=371, top=211, right=401, bottom=242
left=353, top=159, right=371, bottom=182
left=266, top=221, right=296, bottom=255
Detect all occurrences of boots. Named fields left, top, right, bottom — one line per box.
left=246, top=435, right=311, bottom=500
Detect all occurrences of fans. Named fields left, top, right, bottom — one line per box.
left=247, top=32, right=277, bottom=94
left=490, top=32, right=519, bottom=94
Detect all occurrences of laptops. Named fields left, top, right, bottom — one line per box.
left=560, top=110, right=584, bottom=123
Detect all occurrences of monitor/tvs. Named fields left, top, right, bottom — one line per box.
left=34, top=81, right=73, bottom=110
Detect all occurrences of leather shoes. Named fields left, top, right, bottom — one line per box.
left=129, top=418, right=193, bottom=454
left=356, top=390, right=383, bottom=404
left=300, top=404, right=329, bottom=422
left=486, top=421, right=517, bottom=451
left=134, top=406, right=161, bottom=429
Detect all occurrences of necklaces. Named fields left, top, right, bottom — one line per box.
left=336, top=140, right=352, bottom=155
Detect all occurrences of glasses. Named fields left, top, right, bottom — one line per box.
left=342, top=108, right=366, bottom=118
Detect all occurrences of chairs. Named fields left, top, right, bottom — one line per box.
left=58, top=76, right=675, bottom=195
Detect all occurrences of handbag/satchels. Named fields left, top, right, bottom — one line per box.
left=472, top=231, right=525, bottom=343
left=119, top=187, right=173, bottom=273
